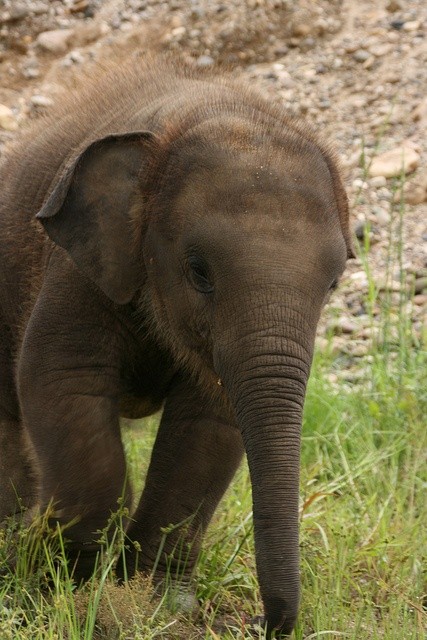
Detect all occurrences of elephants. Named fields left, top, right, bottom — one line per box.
left=1, top=50, right=357, bottom=638
